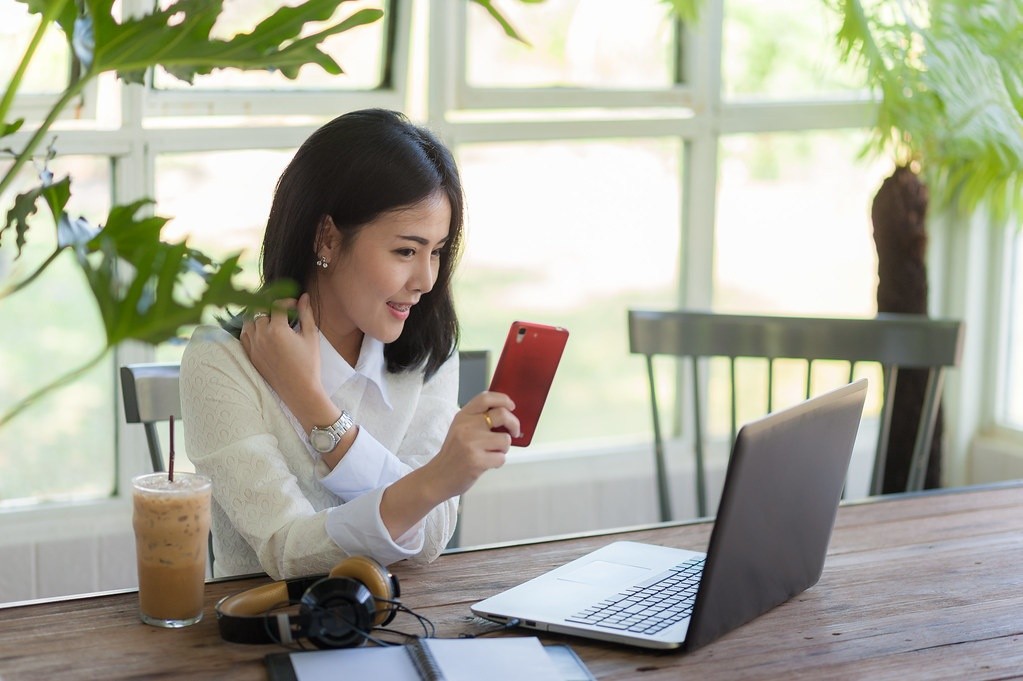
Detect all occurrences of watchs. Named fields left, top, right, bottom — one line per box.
left=307, top=409, right=354, bottom=454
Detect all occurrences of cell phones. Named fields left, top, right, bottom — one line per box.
left=488, top=321, right=569, bottom=448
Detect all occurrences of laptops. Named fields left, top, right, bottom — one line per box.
left=471, top=376, right=870, bottom=655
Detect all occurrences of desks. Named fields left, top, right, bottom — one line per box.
left=0, top=483, right=1023, bottom=681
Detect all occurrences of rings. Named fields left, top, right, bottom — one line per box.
left=483, top=411, right=495, bottom=429
left=253, top=312, right=269, bottom=321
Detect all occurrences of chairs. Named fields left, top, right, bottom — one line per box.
left=626, top=304, right=967, bottom=527
left=119, top=349, right=494, bottom=573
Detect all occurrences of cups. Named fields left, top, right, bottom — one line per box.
left=132, top=472, right=212, bottom=628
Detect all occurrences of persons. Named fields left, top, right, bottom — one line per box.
left=178, top=109, right=519, bottom=582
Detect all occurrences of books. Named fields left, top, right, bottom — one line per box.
left=264, top=636, right=597, bottom=681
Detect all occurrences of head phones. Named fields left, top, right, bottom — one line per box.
left=213, top=555, right=400, bottom=649
